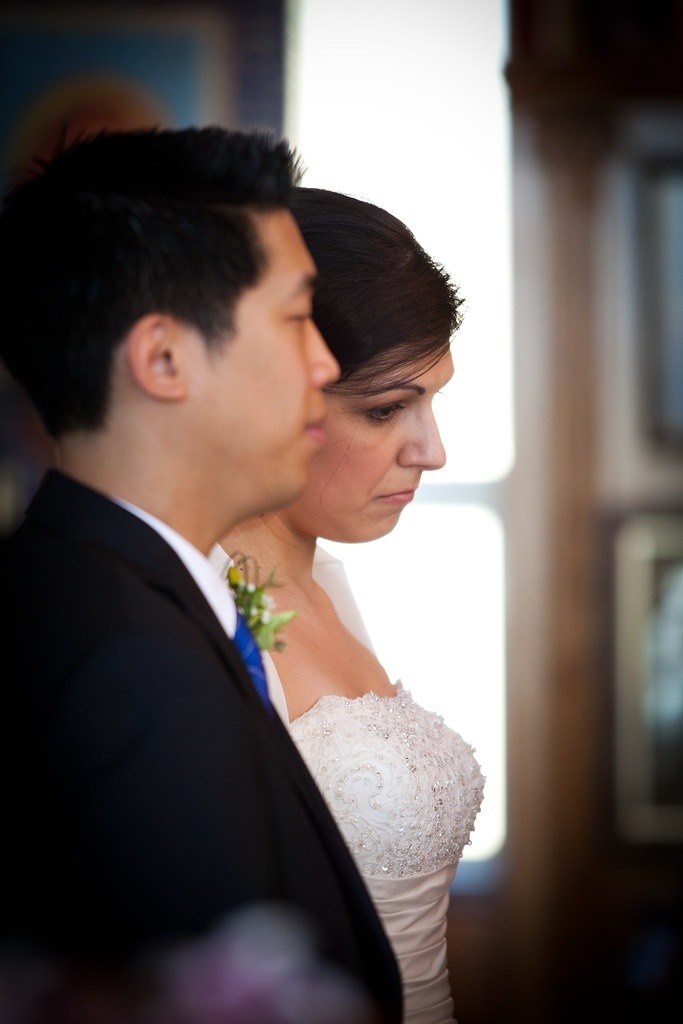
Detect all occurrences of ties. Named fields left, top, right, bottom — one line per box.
left=230, top=615, right=270, bottom=707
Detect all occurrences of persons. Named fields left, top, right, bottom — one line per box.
left=220, top=188, right=486, bottom=1024
left=0, top=126, right=406, bottom=1024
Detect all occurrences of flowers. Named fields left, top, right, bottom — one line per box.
left=224, top=551, right=296, bottom=652
